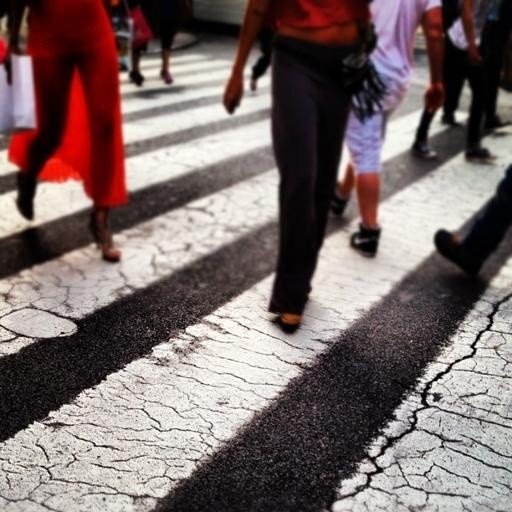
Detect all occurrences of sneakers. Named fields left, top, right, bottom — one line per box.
left=466, top=149, right=489, bottom=159
left=436, top=230, right=481, bottom=276
left=412, top=144, right=438, bottom=159
left=279, top=313, right=301, bottom=331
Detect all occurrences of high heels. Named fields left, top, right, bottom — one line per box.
left=88, top=221, right=120, bottom=262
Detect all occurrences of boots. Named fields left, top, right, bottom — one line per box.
left=352, top=224, right=380, bottom=257
left=328, top=183, right=347, bottom=216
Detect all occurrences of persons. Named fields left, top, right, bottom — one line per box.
left=224, top=0, right=512, bottom=333
left=1, top=0, right=185, bottom=262
